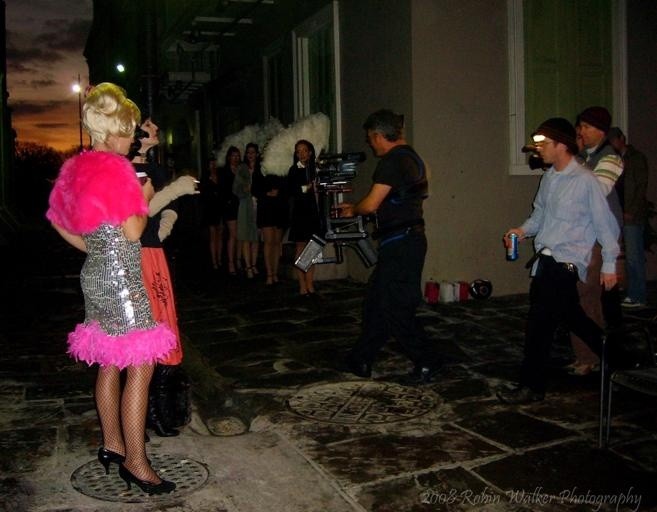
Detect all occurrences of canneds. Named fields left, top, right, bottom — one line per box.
left=505, top=233, right=519, bottom=261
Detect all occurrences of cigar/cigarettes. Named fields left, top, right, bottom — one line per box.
left=193, top=180, right=201, bottom=183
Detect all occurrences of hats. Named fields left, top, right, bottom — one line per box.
left=577, top=106, right=612, bottom=133
left=530, top=117, right=579, bottom=155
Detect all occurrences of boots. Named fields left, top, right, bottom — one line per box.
left=145, top=379, right=180, bottom=438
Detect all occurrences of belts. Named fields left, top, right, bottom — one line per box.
left=524, top=248, right=577, bottom=271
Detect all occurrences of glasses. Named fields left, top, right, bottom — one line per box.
left=532, top=140, right=553, bottom=149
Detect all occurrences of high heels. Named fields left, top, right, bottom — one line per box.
left=97, top=448, right=151, bottom=475
left=117, top=462, right=175, bottom=494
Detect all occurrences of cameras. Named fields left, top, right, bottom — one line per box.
left=520, top=144, right=553, bottom=170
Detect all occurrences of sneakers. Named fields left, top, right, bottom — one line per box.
left=339, top=364, right=372, bottom=380
left=398, top=366, right=437, bottom=385
left=620, top=297, right=646, bottom=307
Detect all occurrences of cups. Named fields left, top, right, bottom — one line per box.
left=136, top=172, right=148, bottom=186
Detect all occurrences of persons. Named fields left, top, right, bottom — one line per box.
left=45, top=80, right=204, bottom=495
left=502, top=103, right=647, bottom=383
left=123, top=107, right=184, bottom=443
left=197, top=138, right=324, bottom=300
left=327, top=108, right=447, bottom=386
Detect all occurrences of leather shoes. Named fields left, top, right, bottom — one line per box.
left=564, top=361, right=599, bottom=374
left=497, top=388, right=544, bottom=405
